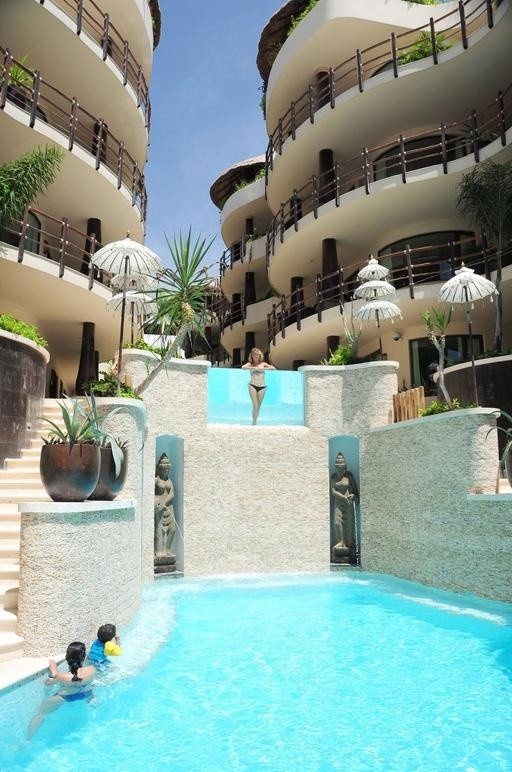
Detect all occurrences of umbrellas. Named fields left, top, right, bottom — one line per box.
left=355, top=252, right=402, bottom=360
left=90, top=228, right=161, bottom=401
left=438, top=259, right=501, bottom=407
left=105, top=271, right=160, bottom=345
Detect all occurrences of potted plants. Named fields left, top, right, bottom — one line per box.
left=0, top=52, right=35, bottom=110
left=29, top=388, right=148, bottom=502
left=458, top=113, right=499, bottom=153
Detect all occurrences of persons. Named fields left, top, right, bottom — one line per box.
left=24, top=642, right=96, bottom=744
left=153, top=452, right=177, bottom=555
left=330, top=452, right=360, bottom=550
left=239, top=347, right=278, bottom=426
left=86, top=624, right=122, bottom=671
